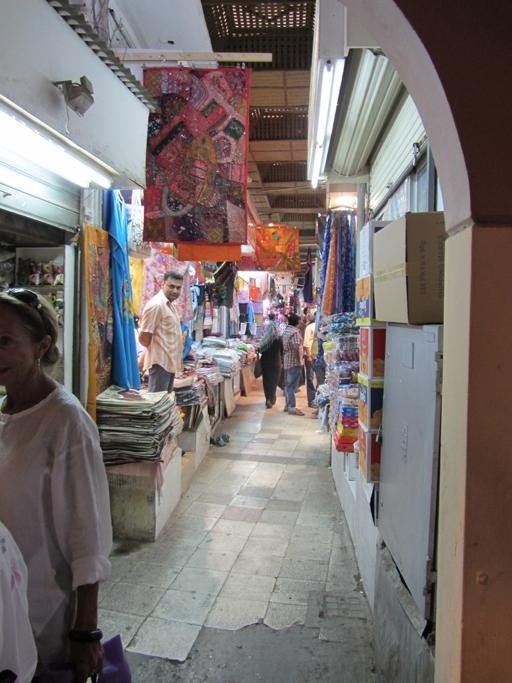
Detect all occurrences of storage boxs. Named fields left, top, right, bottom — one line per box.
left=373, top=211, right=444, bottom=326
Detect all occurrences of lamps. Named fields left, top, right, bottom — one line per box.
left=54, top=75, right=96, bottom=118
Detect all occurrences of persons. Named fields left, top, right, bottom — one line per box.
left=258, top=306, right=318, bottom=416
left=1, top=288, right=113, bottom=682
left=138, top=270, right=184, bottom=392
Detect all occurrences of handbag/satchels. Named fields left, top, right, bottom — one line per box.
left=254, top=352, right=262, bottom=378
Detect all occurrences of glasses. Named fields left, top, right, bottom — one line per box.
left=0, top=287, right=42, bottom=310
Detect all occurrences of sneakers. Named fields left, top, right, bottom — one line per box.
left=284, top=405, right=304, bottom=416
